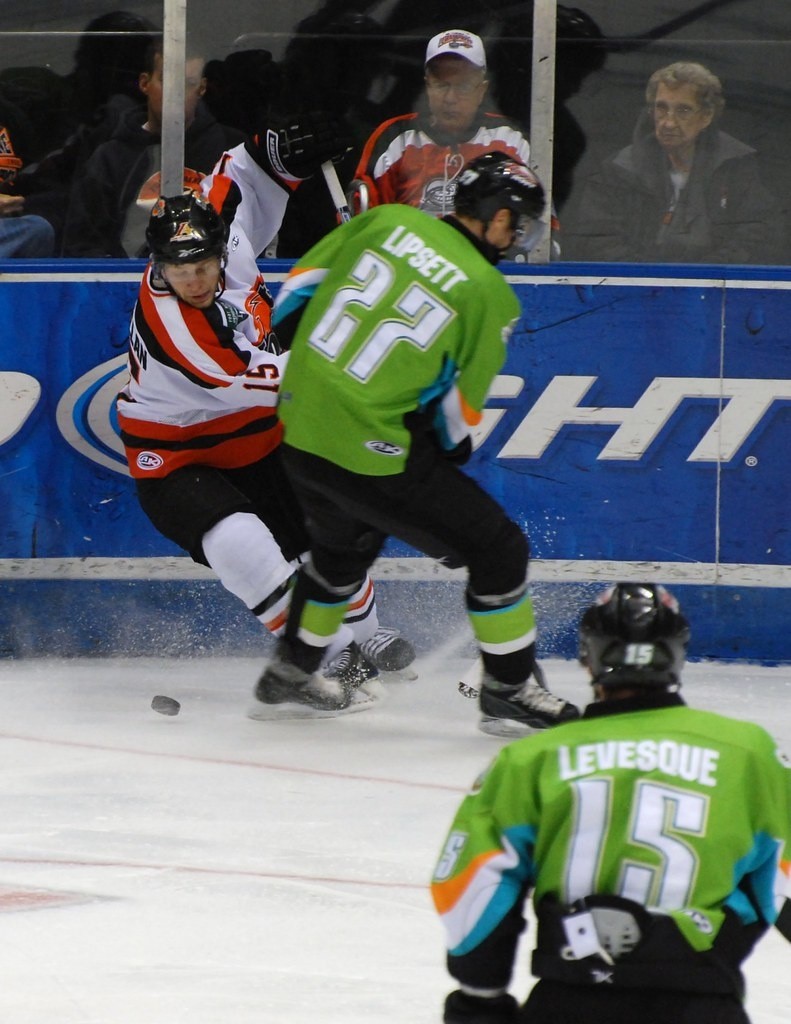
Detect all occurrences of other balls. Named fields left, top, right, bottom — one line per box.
left=151, top=695, right=182, bottom=718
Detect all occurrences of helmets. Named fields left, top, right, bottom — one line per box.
left=145, top=191, right=227, bottom=264
left=577, top=582, right=690, bottom=689
left=453, top=152, right=544, bottom=231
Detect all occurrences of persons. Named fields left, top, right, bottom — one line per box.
left=251, top=151, right=580, bottom=738
left=429, top=584, right=791, bottom=1024
left=0, top=0, right=791, bottom=264
left=117, top=112, right=418, bottom=700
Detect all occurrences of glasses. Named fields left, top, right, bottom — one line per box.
left=647, top=105, right=704, bottom=121
left=419, top=76, right=484, bottom=97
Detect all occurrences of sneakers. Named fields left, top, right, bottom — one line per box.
left=479, top=675, right=579, bottom=740
left=301, top=639, right=378, bottom=712
left=247, top=659, right=378, bottom=720
left=356, top=626, right=417, bottom=671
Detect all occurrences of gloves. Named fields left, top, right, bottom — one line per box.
left=444, top=991, right=520, bottom=1024
left=254, top=108, right=333, bottom=183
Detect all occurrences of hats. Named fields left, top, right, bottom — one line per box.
left=424, top=30, right=486, bottom=68
